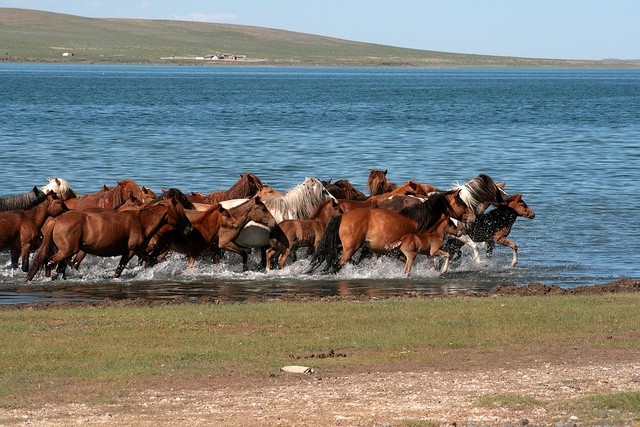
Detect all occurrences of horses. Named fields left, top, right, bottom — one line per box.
left=0, top=166, right=536, bottom=283
